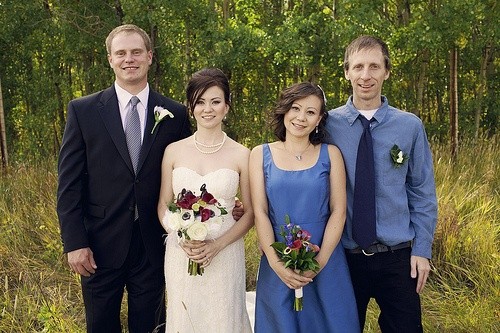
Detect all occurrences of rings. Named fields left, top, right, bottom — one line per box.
left=206, top=257, right=208, bottom=259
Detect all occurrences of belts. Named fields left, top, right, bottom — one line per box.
left=346, top=240, right=411, bottom=256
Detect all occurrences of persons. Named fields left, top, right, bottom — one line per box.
left=249, top=82, right=361, bottom=333
left=56, top=25, right=243, bottom=333
left=318, top=35, right=437, bottom=333
left=158, top=69, right=254, bottom=333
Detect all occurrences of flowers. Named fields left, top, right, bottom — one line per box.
left=167, top=190, right=228, bottom=276
left=150, top=106, right=175, bottom=134
left=390, top=145, right=407, bottom=168
left=269, top=215, right=320, bottom=311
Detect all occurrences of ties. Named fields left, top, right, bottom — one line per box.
left=125, top=96, right=141, bottom=221
left=352, top=114, right=376, bottom=248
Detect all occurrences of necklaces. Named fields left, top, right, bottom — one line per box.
left=282, top=143, right=312, bottom=160
left=194, top=130, right=226, bottom=154
